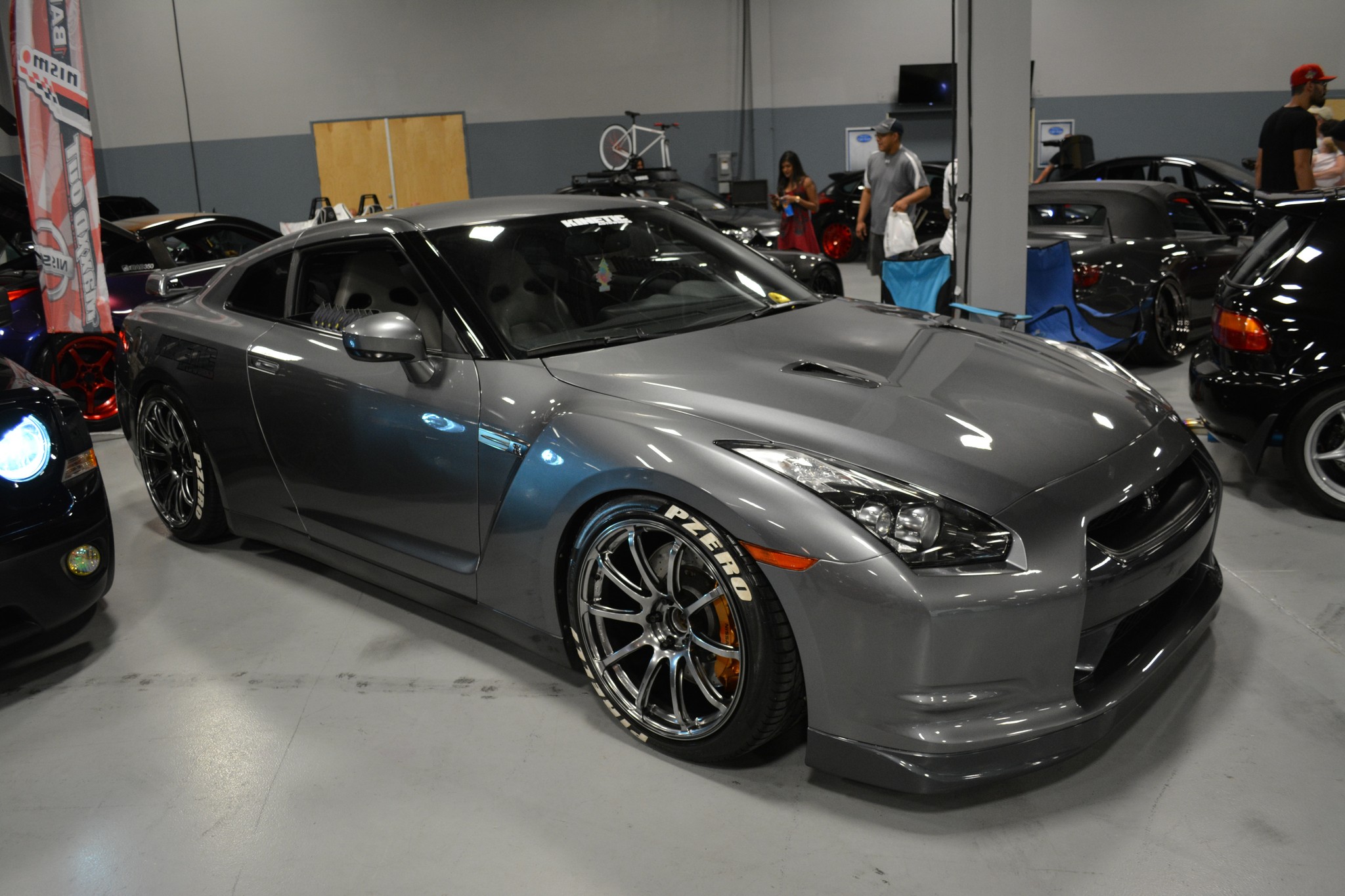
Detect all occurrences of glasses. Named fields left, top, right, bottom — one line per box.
left=1312, top=81, right=1327, bottom=88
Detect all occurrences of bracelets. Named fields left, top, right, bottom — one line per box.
left=796, top=196, right=800, bottom=202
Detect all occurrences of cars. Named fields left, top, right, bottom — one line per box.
left=1060, top=154, right=1261, bottom=237
left=879, top=180, right=1254, bottom=369
left=1, top=354, right=116, bottom=647
left=556, top=171, right=845, bottom=296
left=811, top=160, right=952, bottom=262
left=0, top=171, right=295, bottom=432
left=113, top=193, right=1223, bottom=811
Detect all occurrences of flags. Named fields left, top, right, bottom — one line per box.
left=9, top=2, right=112, bottom=332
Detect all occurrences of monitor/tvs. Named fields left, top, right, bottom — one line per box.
left=897, top=62, right=956, bottom=103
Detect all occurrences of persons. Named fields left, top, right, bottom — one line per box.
left=856, top=118, right=931, bottom=305
left=1257, top=62, right=1336, bottom=193
left=771, top=151, right=820, bottom=254
left=939, top=158, right=958, bottom=260
left=1308, top=107, right=1345, bottom=188
left=1032, top=134, right=1072, bottom=184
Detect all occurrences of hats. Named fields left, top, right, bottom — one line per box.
left=871, top=117, right=904, bottom=136
left=1290, top=63, right=1337, bottom=87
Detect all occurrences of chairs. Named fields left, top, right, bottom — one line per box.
left=482, top=251, right=575, bottom=344
left=334, top=245, right=442, bottom=358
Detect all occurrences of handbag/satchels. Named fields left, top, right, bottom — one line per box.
left=882, top=207, right=919, bottom=259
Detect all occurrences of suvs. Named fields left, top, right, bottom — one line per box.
left=1186, top=183, right=1345, bottom=521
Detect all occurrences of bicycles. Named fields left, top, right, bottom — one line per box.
left=598, top=110, right=680, bottom=171
